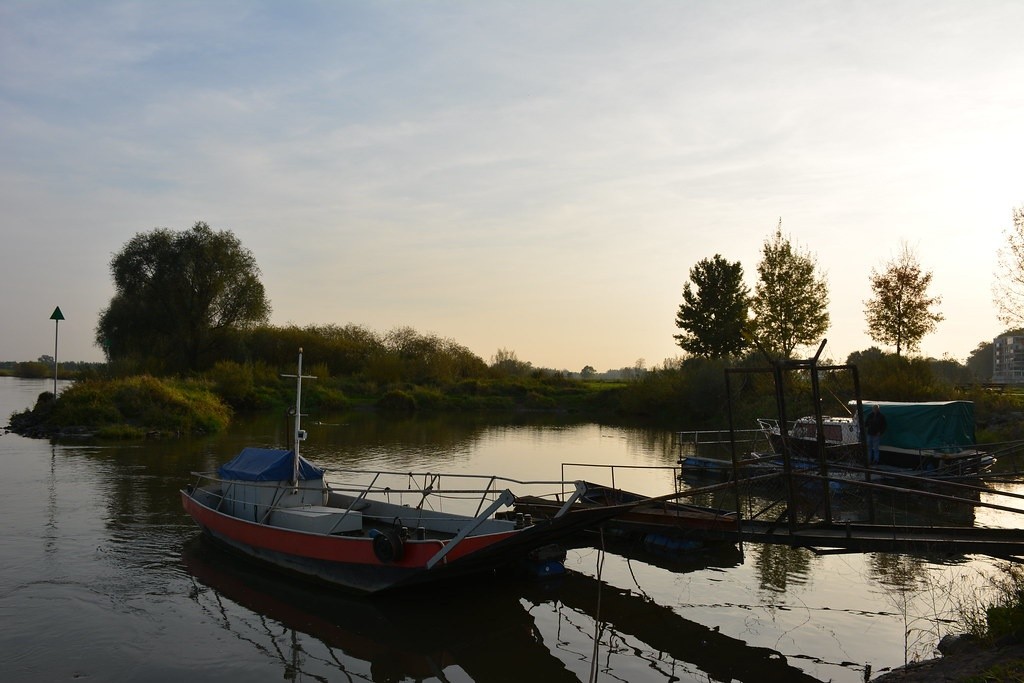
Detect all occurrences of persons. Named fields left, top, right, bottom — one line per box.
left=864, top=404, right=887, bottom=464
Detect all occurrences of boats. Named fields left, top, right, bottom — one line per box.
left=756, top=400, right=997, bottom=474
left=181, top=532, right=534, bottom=683
left=179, top=347, right=588, bottom=597
left=575, top=481, right=743, bottom=519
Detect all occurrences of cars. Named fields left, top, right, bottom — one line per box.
left=986, top=385, right=1003, bottom=393
